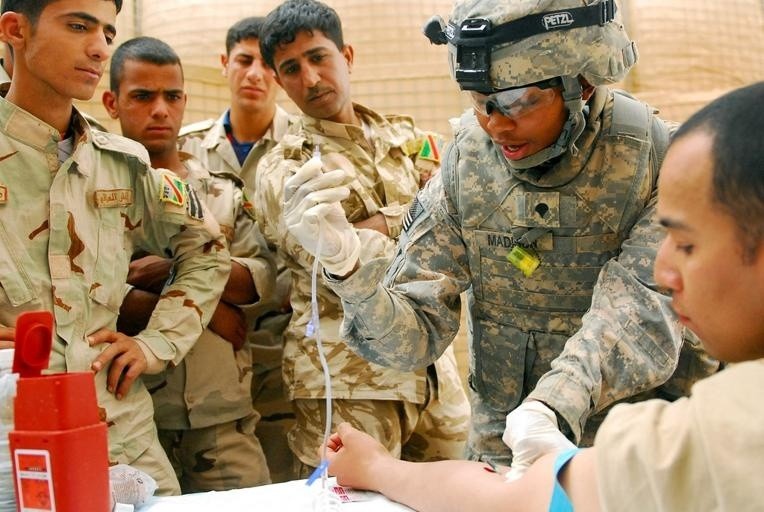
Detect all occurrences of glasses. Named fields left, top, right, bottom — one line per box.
left=467, top=87, right=559, bottom=121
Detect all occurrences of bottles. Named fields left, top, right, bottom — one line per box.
left=7, top=310, right=111, bottom=512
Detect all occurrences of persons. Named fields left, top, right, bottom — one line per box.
left=320, top=83, right=764, bottom=512
left=282, top=1, right=716, bottom=465
left=1, top=1, right=474, bottom=510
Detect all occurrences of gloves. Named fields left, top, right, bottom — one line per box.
left=504, top=400, right=581, bottom=484
left=280, top=157, right=367, bottom=279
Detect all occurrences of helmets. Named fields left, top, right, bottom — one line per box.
left=420, top=0, right=639, bottom=91
